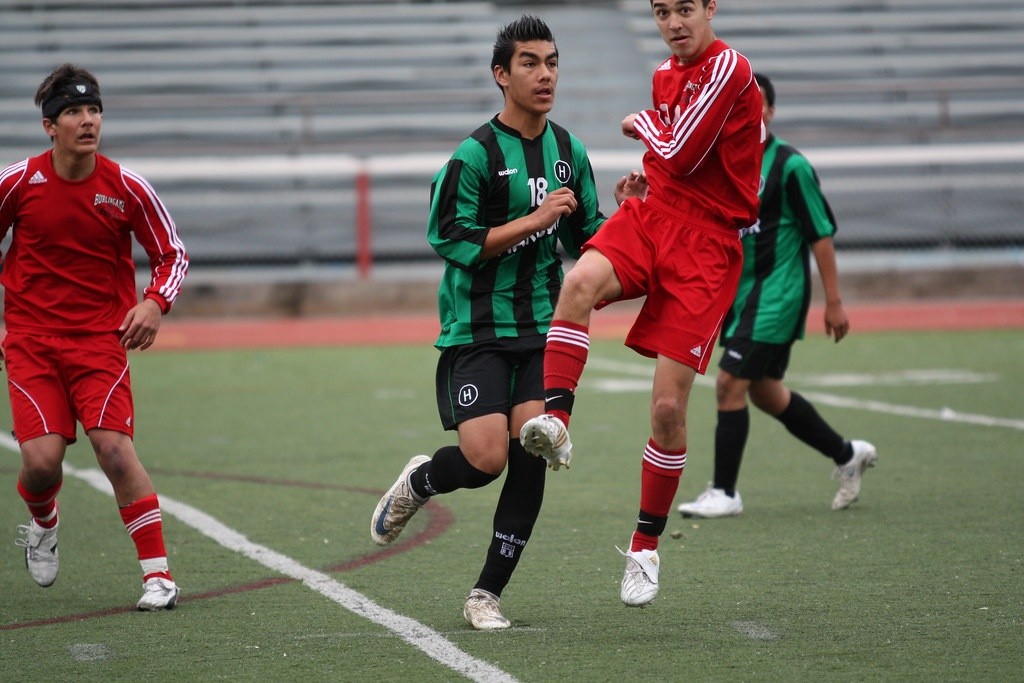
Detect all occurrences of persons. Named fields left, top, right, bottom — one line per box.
left=0, top=61, right=189, bottom=610
left=678, top=72, right=877, bottom=519
left=370, top=16, right=647, bottom=631
left=520, top=0, right=767, bottom=604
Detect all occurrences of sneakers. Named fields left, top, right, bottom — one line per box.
left=14, top=498, right=60, bottom=587
left=829, top=439, right=878, bottom=510
left=676, top=481, right=743, bottom=518
left=464, top=588, right=510, bottom=629
left=136, top=576, right=180, bottom=611
left=520, top=414, right=571, bottom=471
left=371, top=455, right=433, bottom=546
left=614, top=530, right=660, bottom=604
